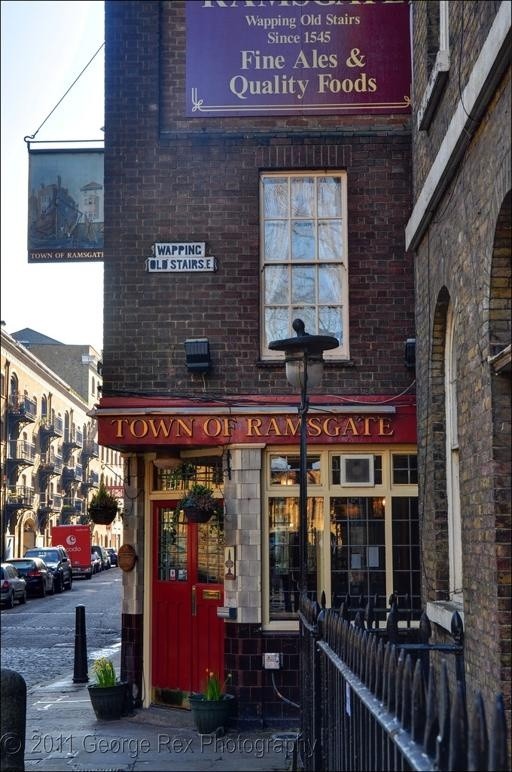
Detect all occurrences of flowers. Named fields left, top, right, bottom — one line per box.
left=181, top=483, right=216, bottom=512
left=93, top=655, right=118, bottom=686
left=204, top=668, right=222, bottom=700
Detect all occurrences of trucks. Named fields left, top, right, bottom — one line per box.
left=52, top=526, right=94, bottom=578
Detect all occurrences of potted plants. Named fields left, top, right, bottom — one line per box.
left=88, top=473, right=117, bottom=524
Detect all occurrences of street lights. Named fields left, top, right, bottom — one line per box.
left=268, top=319, right=339, bottom=596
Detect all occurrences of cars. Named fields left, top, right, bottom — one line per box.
left=0, top=545, right=119, bottom=609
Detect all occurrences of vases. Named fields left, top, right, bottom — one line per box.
left=87, top=680, right=131, bottom=721
left=184, top=509, right=213, bottom=523
left=189, top=692, right=235, bottom=737
